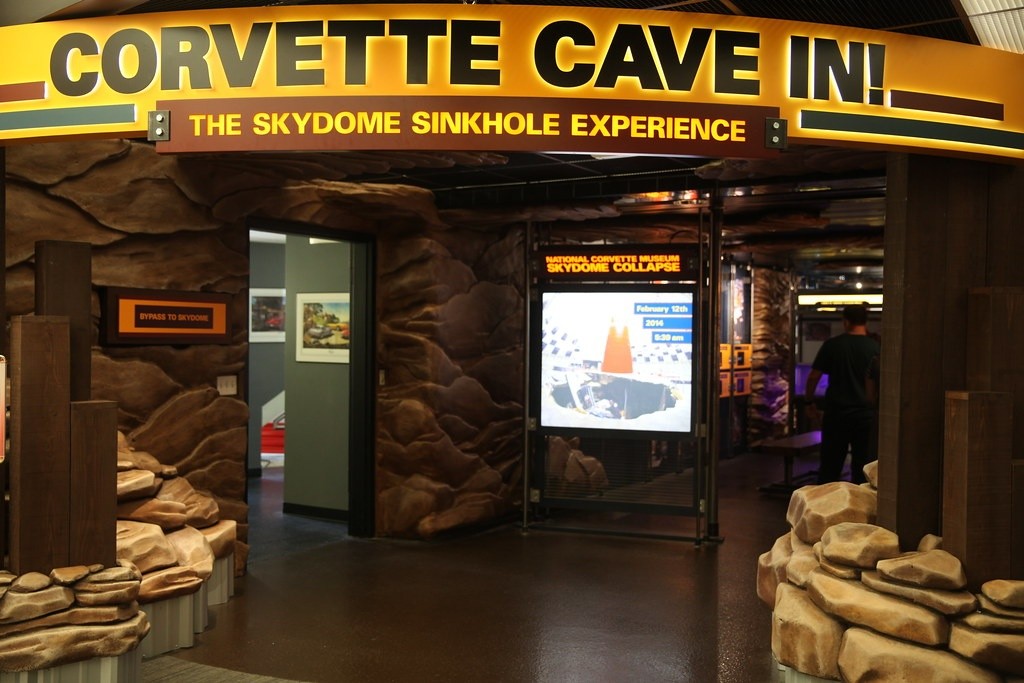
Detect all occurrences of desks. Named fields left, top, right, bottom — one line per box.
left=753, top=429, right=851, bottom=502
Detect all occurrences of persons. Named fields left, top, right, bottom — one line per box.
left=803, top=304, right=881, bottom=485
